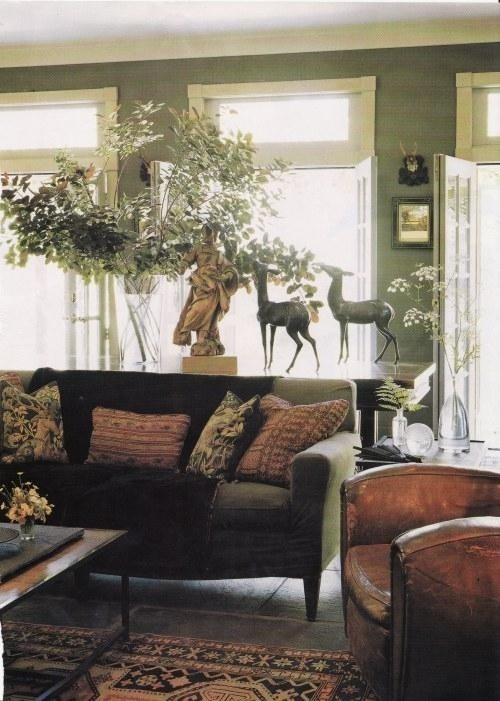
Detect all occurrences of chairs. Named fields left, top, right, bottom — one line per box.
left=340, top=462, right=500, bottom=701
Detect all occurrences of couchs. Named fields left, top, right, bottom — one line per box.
left=0, top=370, right=360, bottom=621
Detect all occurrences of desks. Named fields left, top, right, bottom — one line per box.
left=0, top=356, right=435, bottom=453
left=355, top=435, right=487, bottom=474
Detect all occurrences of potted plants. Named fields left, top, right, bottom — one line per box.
left=376, top=376, right=430, bottom=450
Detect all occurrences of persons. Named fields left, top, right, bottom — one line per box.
left=174, top=223, right=240, bottom=357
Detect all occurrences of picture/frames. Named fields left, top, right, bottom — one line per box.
left=392, top=196, right=434, bottom=250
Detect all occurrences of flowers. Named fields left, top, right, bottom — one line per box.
left=388, top=247, right=487, bottom=423
left=0, top=471, right=55, bottom=535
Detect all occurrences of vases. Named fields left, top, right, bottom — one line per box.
left=438, top=375, right=470, bottom=453
left=19, top=517, right=34, bottom=540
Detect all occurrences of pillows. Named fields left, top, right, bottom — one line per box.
left=0, top=375, right=70, bottom=464
left=84, top=406, right=191, bottom=474
left=0, top=372, right=25, bottom=394
left=234, top=393, right=350, bottom=489
left=185, top=390, right=264, bottom=483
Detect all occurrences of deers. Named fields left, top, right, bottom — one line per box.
left=321, top=264, right=399, bottom=365
left=253, top=261, right=320, bottom=373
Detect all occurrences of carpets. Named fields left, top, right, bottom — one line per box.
left=0, top=622, right=383, bottom=701
